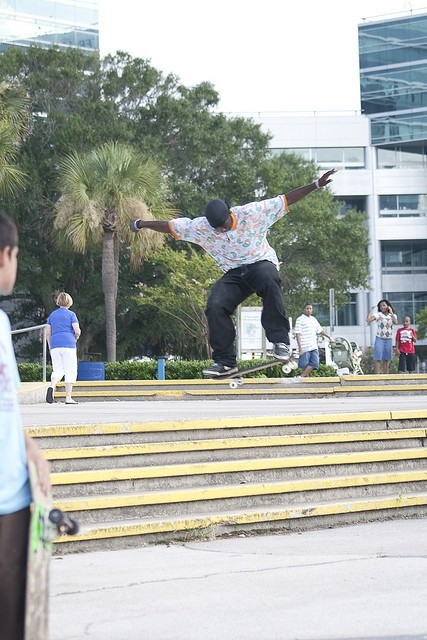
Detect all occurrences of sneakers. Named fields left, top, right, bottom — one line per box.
left=202, top=362, right=238, bottom=376
left=273, top=341, right=291, bottom=360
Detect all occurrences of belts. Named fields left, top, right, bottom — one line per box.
left=401, top=352, right=414, bottom=356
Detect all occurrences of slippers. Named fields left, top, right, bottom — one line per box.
left=65, top=400, right=77, bottom=404
left=46, top=387, right=53, bottom=404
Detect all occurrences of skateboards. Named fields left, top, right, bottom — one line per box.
left=24, top=461, right=80, bottom=640
left=211, top=353, right=299, bottom=389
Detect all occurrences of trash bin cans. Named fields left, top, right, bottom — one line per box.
left=78, top=352, right=105, bottom=381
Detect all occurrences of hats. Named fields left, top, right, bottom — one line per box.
left=206, top=199, right=229, bottom=229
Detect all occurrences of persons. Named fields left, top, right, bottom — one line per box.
left=395, top=315, right=417, bottom=374
left=1, top=210, right=51, bottom=640
left=45, top=292, right=80, bottom=405
left=366, top=300, right=398, bottom=374
left=130, top=168, right=337, bottom=374
left=294, top=304, right=333, bottom=379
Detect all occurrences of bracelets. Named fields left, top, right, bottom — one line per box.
left=313, top=178, right=320, bottom=193
left=134, top=218, right=141, bottom=234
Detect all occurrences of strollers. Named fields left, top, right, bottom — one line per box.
left=328, top=336, right=365, bottom=376
